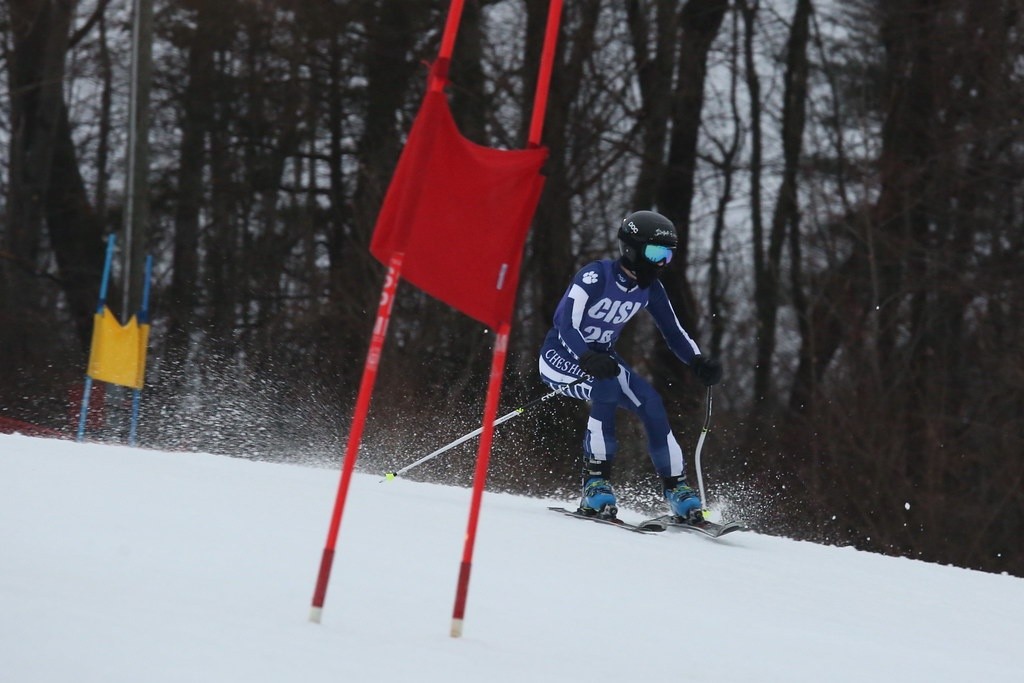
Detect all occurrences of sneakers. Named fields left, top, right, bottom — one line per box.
left=662, top=474, right=702, bottom=517
left=582, top=459, right=616, bottom=511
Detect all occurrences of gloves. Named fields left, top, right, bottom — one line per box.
left=580, top=350, right=617, bottom=379
left=692, top=355, right=722, bottom=385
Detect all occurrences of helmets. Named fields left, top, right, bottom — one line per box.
left=619, top=210, right=678, bottom=278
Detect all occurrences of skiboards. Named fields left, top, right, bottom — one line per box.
left=547, top=506, right=740, bottom=538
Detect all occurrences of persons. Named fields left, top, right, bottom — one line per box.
left=539, top=209, right=721, bottom=517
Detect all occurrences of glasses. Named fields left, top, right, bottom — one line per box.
left=643, top=242, right=674, bottom=265
